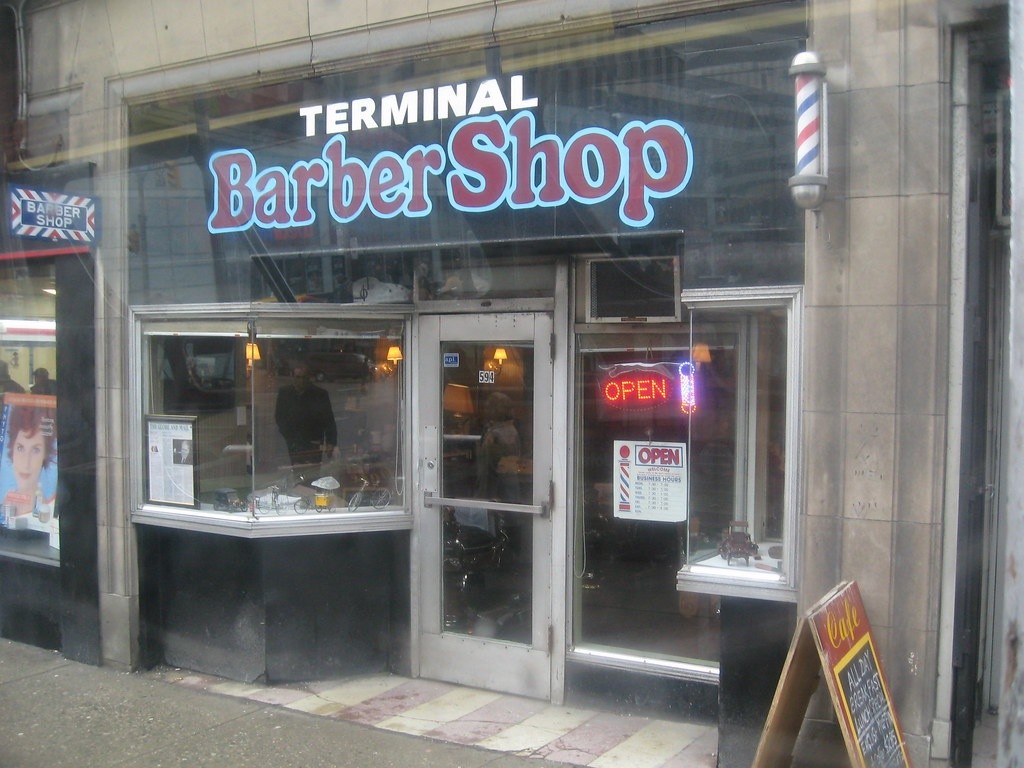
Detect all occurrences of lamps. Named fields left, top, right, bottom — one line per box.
left=380, top=347, right=403, bottom=379
left=492, top=348, right=507, bottom=375
left=246, top=343, right=261, bottom=368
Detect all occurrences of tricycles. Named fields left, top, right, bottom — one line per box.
left=315, top=488, right=336, bottom=513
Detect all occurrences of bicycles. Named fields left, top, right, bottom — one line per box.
left=258, top=485, right=290, bottom=516
left=294, top=490, right=323, bottom=514
left=347, top=477, right=392, bottom=511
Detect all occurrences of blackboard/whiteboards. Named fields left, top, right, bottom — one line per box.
left=808, top=584, right=909, bottom=768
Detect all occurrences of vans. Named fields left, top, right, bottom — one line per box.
left=306, top=351, right=378, bottom=380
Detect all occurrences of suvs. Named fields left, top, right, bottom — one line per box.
left=213, top=486, right=248, bottom=513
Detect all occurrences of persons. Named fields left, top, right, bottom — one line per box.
left=361, top=357, right=369, bottom=384
left=275, top=361, right=337, bottom=486
left=165, top=337, right=193, bottom=410
left=30, top=369, right=59, bottom=392
left=1, top=403, right=55, bottom=519
left=0, top=360, right=23, bottom=392
left=472, top=392, right=521, bottom=500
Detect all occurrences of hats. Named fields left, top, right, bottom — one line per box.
left=32, top=368, right=49, bottom=377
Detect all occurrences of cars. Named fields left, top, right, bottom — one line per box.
left=180, top=354, right=239, bottom=406
